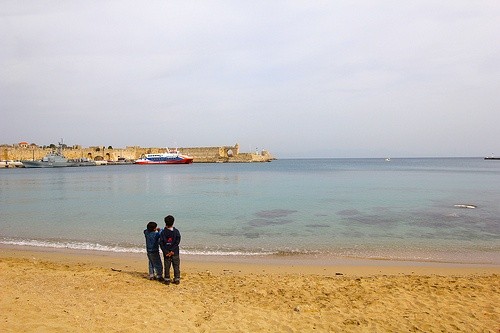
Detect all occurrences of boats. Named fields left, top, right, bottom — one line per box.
left=0, top=158, right=136, bottom=168
left=136, top=146, right=194, bottom=165
left=20, top=138, right=97, bottom=168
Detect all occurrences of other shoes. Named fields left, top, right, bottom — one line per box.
left=162, top=278, right=170, bottom=285
left=157, top=277, right=164, bottom=281
left=150, top=276, right=157, bottom=280
left=171, top=278, right=179, bottom=285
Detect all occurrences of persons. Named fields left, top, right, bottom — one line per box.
left=160, top=216, right=181, bottom=285
left=144, top=222, right=165, bottom=281
